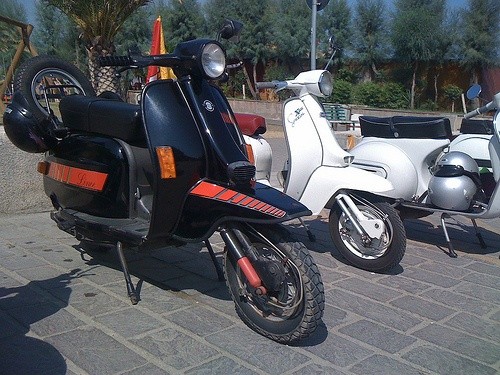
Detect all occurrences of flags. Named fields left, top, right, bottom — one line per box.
left=145, top=16, right=177, bottom=85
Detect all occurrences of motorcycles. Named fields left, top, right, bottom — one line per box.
left=350, top=85, right=500, bottom=258
left=217, top=33, right=405, bottom=273
left=11, top=19, right=324, bottom=342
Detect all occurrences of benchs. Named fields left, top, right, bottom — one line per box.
left=322, top=102, right=355, bottom=131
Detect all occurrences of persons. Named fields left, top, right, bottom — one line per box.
left=130, top=71, right=142, bottom=89
